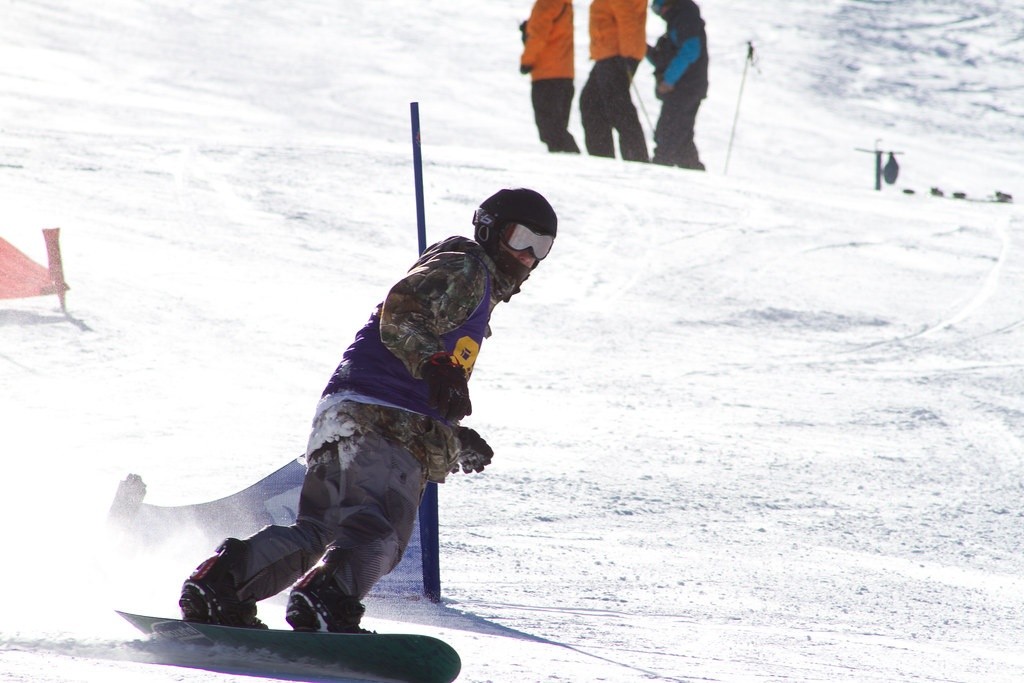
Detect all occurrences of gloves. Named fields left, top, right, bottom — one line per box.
left=446, top=427, right=494, bottom=474
left=520, top=22, right=527, bottom=31
left=426, top=364, right=473, bottom=420
left=520, top=65, right=532, bottom=75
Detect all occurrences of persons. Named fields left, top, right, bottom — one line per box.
left=520, top=0, right=581, bottom=153
left=580, top=0, right=649, bottom=163
left=180, top=188, right=558, bottom=634
left=646, top=0, right=708, bottom=169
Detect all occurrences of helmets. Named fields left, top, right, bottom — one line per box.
left=474, top=188, right=558, bottom=285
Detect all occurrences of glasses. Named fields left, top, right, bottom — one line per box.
left=496, top=219, right=554, bottom=260
left=651, top=0, right=665, bottom=13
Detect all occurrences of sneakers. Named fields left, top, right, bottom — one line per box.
left=285, top=569, right=374, bottom=633
left=179, top=536, right=269, bottom=630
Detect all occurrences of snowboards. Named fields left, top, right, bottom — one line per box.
left=114, top=609, right=461, bottom=683
